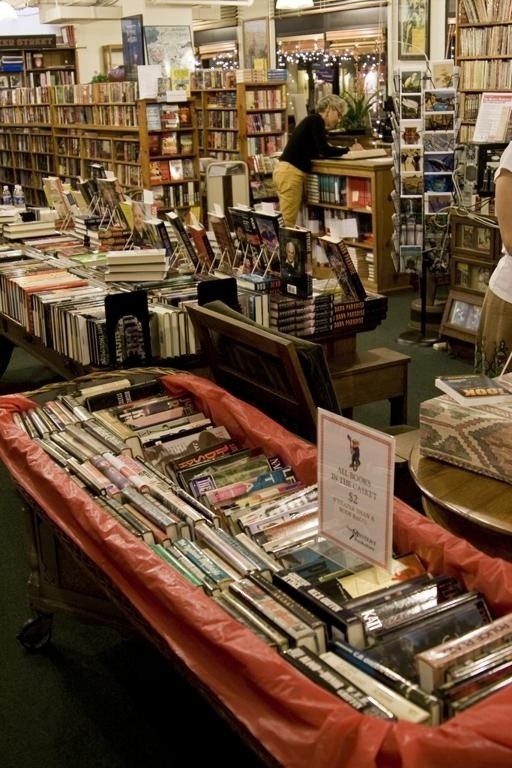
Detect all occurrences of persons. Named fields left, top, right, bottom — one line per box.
left=283, top=241, right=298, bottom=275
left=271, top=94, right=363, bottom=225
left=262, top=224, right=276, bottom=241
left=471, top=135, right=512, bottom=368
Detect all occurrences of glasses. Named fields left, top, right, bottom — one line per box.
left=334, top=106, right=345, bottom=122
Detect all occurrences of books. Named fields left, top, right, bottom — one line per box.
left=306, top=207, right=359, bottom=239
left=464, top=94, right=482, bottom=120
left=278, top=225, right=313, bottom=299
left=61, top=24, right=79, bottom=47
left=248, top=136, right=283, bottom=155
left=206, top=131, right=239, bottom=150
left=306, top=174, right=362, bottom=208
left=457, top=0, right=512, bottom=22
left=58, top=157, right=79, bottom=174
left=156, top=64, right=193, bottom=103
left=312, top=241, right=326, bottom=265
left=235, top=274, right=282, bottom=290
left=208, top=111, right=238, bottom=128
left=14, top=372, right=511, bottom=732
left=29, top=71, right=76, bottom=86
left=367, top=252, right=374, bottom=281
left=246, top=89, right=282, bottom=108
left=209, top=152, right=239, bottom=161
left=114, top=142, right=139, bottom=161
left=0, top=151, right=53, bottom=172
left=0, top=87, right=49, bottom=106
left=149, top=132, right=192, bottom=157
left=472, top=93, right=512, bottom=143
left=346, top=244, right=362, bottom=275
left=55, top=106, right=137, bottom=127
left=190, top=69, right=234, bottom=88
left=83, top=139, right=112, bottom=158
left=114, top=164, right=142, bottom=186
left=252, top=177, right=276, bottom=198
left=250, top=155, right=275, bottom=171
left=145, top=104, right=191, bottom=131
left=186, top=223, right=219, bottom=272
left=0, top=127, right=50, bottom=134
left=247, top=112, right=281, bottom=132
left=253, top=205, right=282, bottom=274
left=460, top=124, right=474, bottom=141
left=53, top=80, right=136, bottom=104
left=149, top=160, right=195, bottom=181
left=311, top=277, right=343, bottom=295
left=235, top=56, right=288, bottom=82
left=153, top=183, right=200, bottom=207
left=0, top=134, right=53, bottom=152
left=186, top=289, right=390, bottom=342
left=25, top=52, right=45, bottom=68
left=229, top=203, right=265, bottom=268
left=0, top=174, right=186, bottom=371
left=0, top=107, right=50, bottom=123
left=458, top=26, right=512, bottom=56
left=207, top=204, right=240, bottom=265
left=458, top=59, right=512, bottom=90
left=0, top=73, right=24, bottom=87
left=56, top=138, right=79, bottom=156
left=66, top=128, right=134, bottom=139
left=317, top=234, right=366, bottom=301
left=205, top=92, right=235, bottom=107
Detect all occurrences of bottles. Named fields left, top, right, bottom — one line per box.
left=16, top=186, right=25, bottom=208
left=2, top=185, right=11, bottom=205
left=12, top=184, right=20, bottom=205
